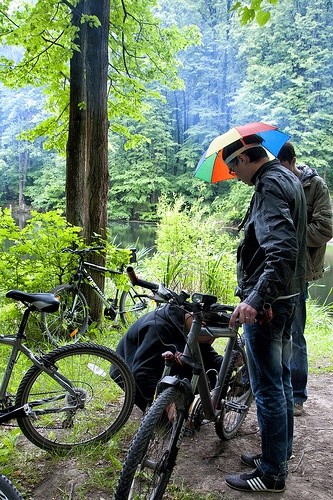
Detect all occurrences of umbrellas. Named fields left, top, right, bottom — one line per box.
left=192, top=121, right=290, bottom=184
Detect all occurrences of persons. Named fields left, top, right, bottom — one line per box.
left=109, top=297, right=245, bottom=432
left=221, top=134, right=308, bottom=493
left=276, top=143, right=333, bottom=416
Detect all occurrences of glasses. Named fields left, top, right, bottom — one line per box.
left=229, top=160, right=239, bottom=176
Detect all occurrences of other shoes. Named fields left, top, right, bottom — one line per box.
left=293, top=403, right=304, bottom=416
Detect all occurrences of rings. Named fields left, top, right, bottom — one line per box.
left=245, top=317, right=249, bottom=319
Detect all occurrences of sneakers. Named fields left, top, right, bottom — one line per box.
left=240, top=452, right=289, bottom=477
left=225, top=464, right=286, bottom=494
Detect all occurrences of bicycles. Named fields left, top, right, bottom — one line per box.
left=114, top=267, right=254, bottom=500
left=0, top=290, right=137, bottom=500
left=41, top=246, right=160, bottom=346
